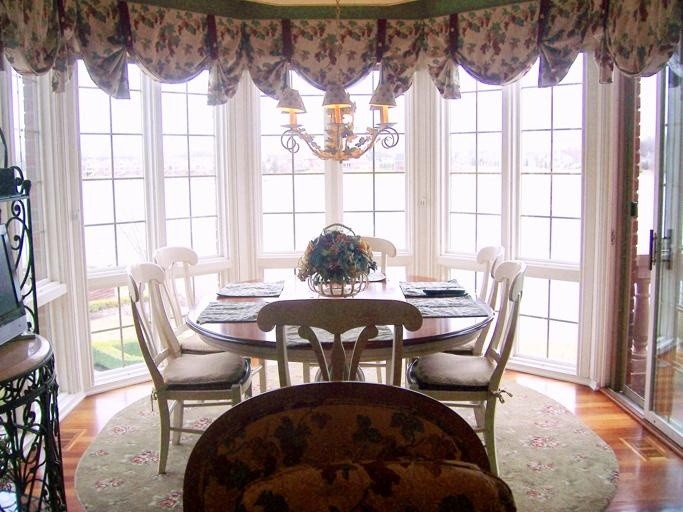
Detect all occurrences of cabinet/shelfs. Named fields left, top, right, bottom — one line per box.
left=0, top=333, right=68, bottom=512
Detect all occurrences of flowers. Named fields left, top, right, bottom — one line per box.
left=293, top=234, right=376, bottom=281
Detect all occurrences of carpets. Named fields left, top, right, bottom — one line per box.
left=74, top=376, right=619, bottom=511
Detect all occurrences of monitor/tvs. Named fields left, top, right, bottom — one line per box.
left=0, top=224, right=27, bottom=346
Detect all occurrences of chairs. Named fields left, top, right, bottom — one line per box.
left=407, top=261, right=527, bottom=477
left=303, top=236, right=397, bottom=384
left=257, top=299, right=423, bottom=387
left=441, top=245, right=504, bottom=428
left=127, top=263, right=253, bottom=474
left=154, top=245, right=266, bottom=394
left=276, top=1, right=399, bottom=164
left=184, top=381, right=490, bottom=511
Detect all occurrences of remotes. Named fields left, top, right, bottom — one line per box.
left=423, top=288, right=465, bottom=295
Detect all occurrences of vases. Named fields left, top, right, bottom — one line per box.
left=307, top=223, right=370, bottom=298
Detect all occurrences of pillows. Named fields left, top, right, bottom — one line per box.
left=241, top=456, right=516, bottom=511
